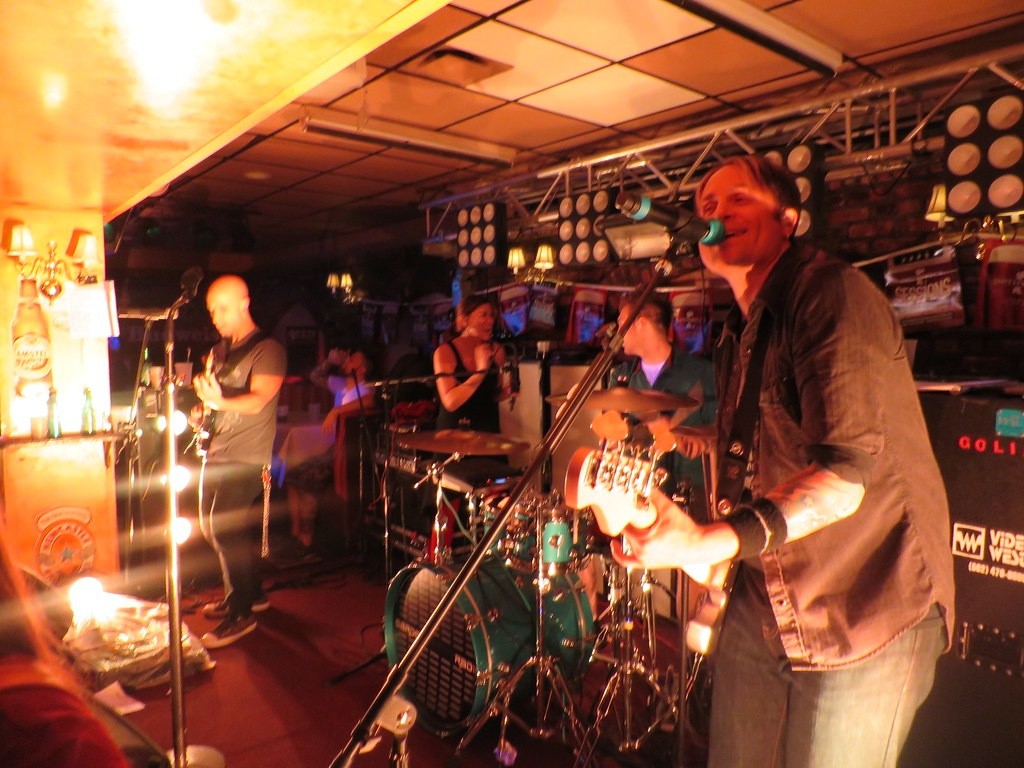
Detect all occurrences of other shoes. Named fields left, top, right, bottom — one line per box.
left=202, top=595, right=269, bottom=617
left=202, top=612, right=258, bottom=649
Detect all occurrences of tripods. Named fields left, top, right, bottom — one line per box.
left=455, top=525, right=707, bottom=768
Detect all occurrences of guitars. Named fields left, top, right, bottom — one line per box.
left=196, top=347, right=218, bottom=456
left=560, top=411, right=741, bottom=657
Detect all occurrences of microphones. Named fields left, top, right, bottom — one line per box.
left=616, top=193, right=726, bottom=246
left=510, top=349, right=520, bottom=399
left=118, top=307, right=178, bottom=320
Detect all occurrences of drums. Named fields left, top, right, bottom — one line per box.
left=380, top=538, right=598, bottom=731
left=469, top=490, right=592, bottom=580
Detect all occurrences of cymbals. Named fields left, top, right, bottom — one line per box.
left=371, top=450, right=474, bottom=494
left=394, top=428, right=531, bottom=455
left=541, top=385, right=698, bottom=410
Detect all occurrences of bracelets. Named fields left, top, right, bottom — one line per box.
left=724, top=505, right=766, bottom=560
left=752, top=498, right=787, bottom=551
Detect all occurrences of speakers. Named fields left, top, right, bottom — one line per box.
left=87, top=694, right=171, bottom=768
left=375, top=447, right=460, bottom=538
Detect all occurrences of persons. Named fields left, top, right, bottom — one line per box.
left=308, top=345, right=374, bottom=550
left=0, top=514, right=137, bottom=768
left=419, top=293, right=510, bottom=532
left=611, top=155, right=956, bottom=768
left=609, top=281, right=718, bottom=493
left=186, top=274, right=287, bottom=649
